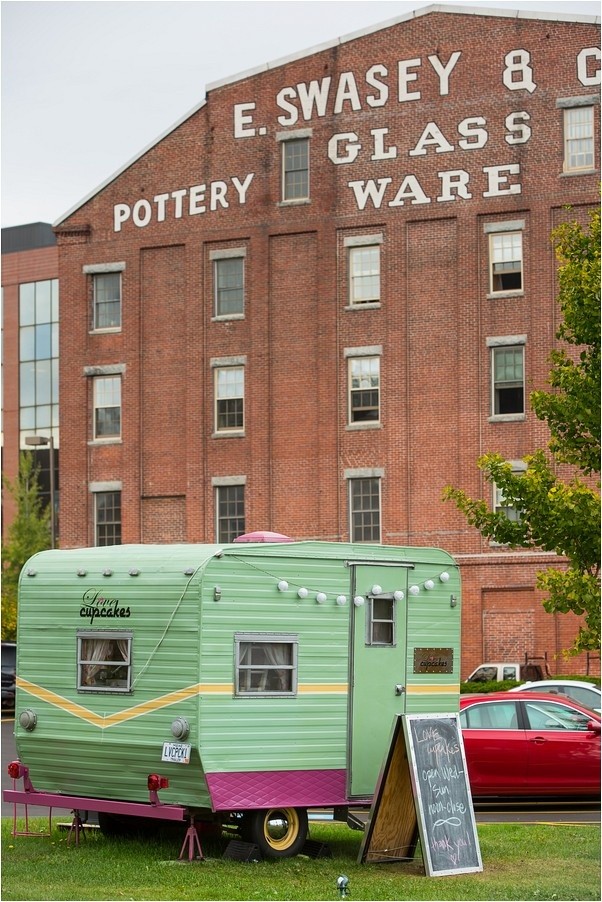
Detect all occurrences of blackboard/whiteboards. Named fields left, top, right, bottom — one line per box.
left=357, top=712, right=483, bottom=877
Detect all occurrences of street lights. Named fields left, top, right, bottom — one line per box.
left=25, top=436, right=55, bottom=549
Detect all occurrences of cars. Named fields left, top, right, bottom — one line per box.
left=503, top=679, right=601, bottom=715
left=460, top=692, right=601, bottom=802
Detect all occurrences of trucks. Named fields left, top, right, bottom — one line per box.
left=462, top=663, right=542, bottom=682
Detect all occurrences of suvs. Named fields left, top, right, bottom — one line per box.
left=1, top=640, right=16, bottom=716
